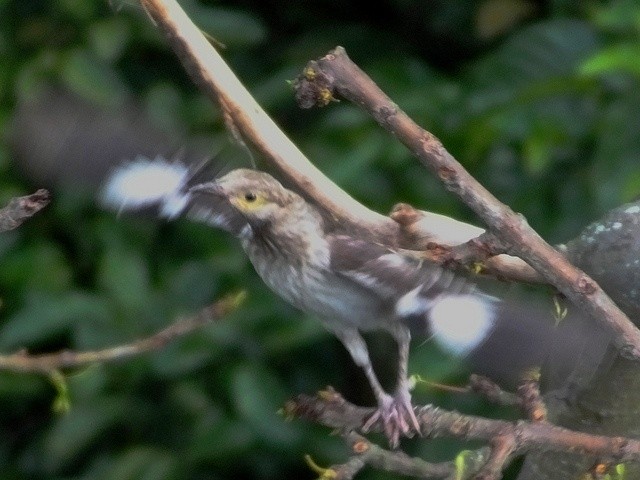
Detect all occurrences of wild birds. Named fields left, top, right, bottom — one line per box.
left=100, top=150, right=611, bottom=450
